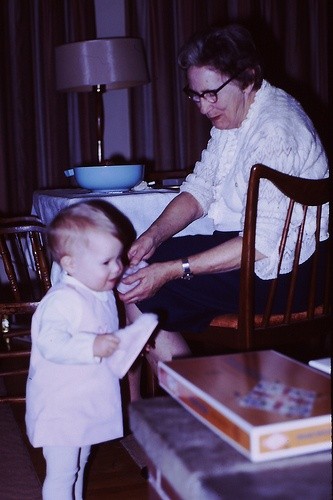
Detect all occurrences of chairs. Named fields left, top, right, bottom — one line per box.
left=146, top=163, right=333, bottom=397
left=0, top=216, right=52, bottom=404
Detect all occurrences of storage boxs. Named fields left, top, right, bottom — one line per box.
left=157, top=350, right=332, bottom=464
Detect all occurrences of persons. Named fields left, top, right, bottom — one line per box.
left=117, top=22, right=330, bottom=402
left=24, top=202, right=123, bottom=500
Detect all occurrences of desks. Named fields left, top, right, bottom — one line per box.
left=24, top=189, right=214, bottom=271
left=129, top=399, right=333, bottom=500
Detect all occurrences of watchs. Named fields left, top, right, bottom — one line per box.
left=181, top=257, right=193, bottom=281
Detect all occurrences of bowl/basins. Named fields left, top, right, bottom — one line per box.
left=74, top=165, right=145, bottom=193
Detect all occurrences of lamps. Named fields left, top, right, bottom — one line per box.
left=54, top=38, right=152, bottom=165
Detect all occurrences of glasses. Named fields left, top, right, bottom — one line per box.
left=182, top=66, right=248, bottom=104
